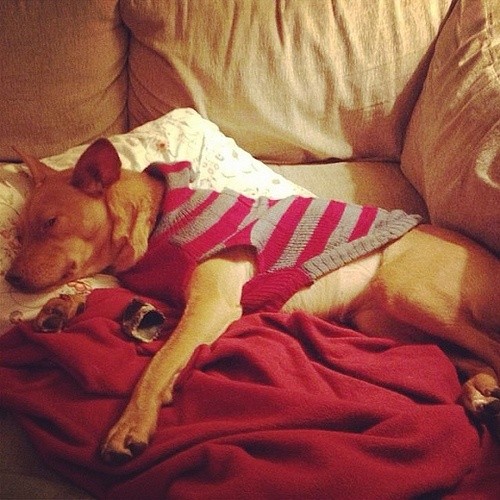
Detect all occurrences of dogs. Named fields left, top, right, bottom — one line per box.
left=4, top=138, right=500, bottom=467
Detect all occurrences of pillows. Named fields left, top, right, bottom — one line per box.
left=397, top=0, right=499, bottom=255
left=1, top=103, right=316, bottom=343
left=116, top=0, right=457, bottom=165
left=0, top=0, right=129, bottom=162
